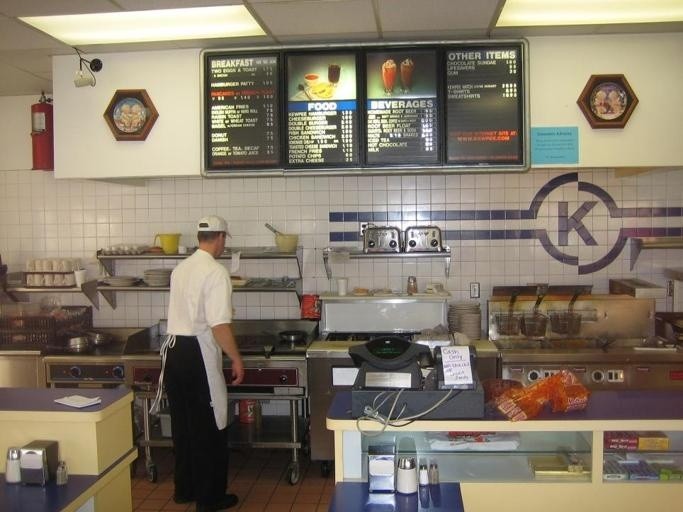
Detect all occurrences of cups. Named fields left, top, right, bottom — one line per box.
left=26, top=258, right=87, bottom=288
left=338, top=279, right=347, bottom=296
left=383, top=60, right=414, bottom=96
left=328, top=63, right=340, bottom=89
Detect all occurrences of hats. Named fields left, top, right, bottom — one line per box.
left=197, top=214, right=233, bottom=237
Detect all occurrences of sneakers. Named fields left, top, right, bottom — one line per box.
left=173, top=494, right=239, bottom=511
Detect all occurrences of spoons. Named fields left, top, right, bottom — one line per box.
left=298, top=82, right=313, bottom=103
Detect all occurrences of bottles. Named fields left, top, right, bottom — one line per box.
left=6, top=446, right=22, bottom=482
left=56, top=462, right=68, bottom=486
left=407, top=276, right=417, bottom=294
left=397, top=455, right=439, bottom=494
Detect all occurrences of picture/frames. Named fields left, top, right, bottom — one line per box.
left=104, top=90, right=157, bottom=140
left=575, top=73, right=639, bottom=129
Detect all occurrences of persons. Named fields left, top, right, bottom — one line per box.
left=148, top=213, right=246, bottom=512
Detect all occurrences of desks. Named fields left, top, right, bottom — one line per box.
left=329, top=468, right=683, bottom=512
left=0, top=385, right=134, bottom=512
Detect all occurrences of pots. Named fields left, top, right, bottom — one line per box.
left=47, top=325, right=111, bottom=352
left=262, top=330, right=305, bottom=341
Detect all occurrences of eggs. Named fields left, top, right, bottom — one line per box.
left=105, top=243, right=140, bottom=252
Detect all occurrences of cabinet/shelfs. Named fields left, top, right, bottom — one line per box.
left=0, top=244, right=304, bottom=309
left=325, top=389, right=683, bottom=482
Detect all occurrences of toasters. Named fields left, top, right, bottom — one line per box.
left=363, top=226, right=443, bottom=254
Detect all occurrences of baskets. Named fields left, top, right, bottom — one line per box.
left=0, top=306, right=92, bottom=348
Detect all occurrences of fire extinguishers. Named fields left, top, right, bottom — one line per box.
left=31, top=91, right=53, bottom=170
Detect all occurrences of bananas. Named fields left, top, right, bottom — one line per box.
left=311, top=81, right=334, bottom=100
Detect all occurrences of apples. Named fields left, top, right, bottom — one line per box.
left=120, top=119, right=142, bottom=128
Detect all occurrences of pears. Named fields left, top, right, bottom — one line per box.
left=121, top=104, right=130, bottom=114
left=131, top=103, right=141, bottom=115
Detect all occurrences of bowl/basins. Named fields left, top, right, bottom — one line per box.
left=276, top=234, right=298, bottom=252
left=304, top=72, right=317, bottom=84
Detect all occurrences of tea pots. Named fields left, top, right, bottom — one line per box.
left=154, top=233, right=182, bottom=255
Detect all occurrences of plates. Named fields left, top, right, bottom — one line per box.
left=114, top=97, right=146, bottom=134
left=448, top=303, right=482, bottom=341
left=590, top=80, right=628, bottom=121
left=103, top=269, right=170, bottom=286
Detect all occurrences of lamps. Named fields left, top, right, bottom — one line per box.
left=73, top=46, right=103, bottom=88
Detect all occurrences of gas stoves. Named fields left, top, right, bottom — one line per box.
left=124, top=334, right=314, bottom=400
